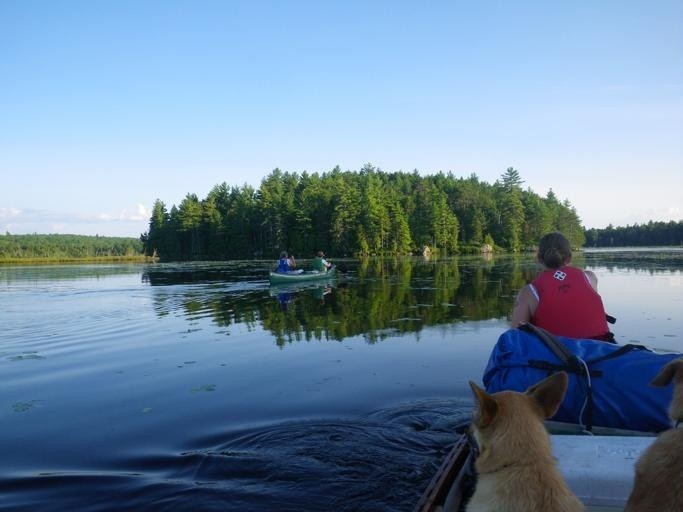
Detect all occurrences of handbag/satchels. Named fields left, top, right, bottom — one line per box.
left=481, top=323, right=683, bottom=435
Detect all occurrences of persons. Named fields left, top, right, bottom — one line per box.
left=312, top=250, right=330, bottom=273
left=270, top=250, right=303, bottom=274
left=312, top=284, right=331, bottom=306
left=512, top=232, right=615, bottom=343
left=276, top=292, right=296, bottom=311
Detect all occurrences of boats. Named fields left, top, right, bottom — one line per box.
left=268, top=265, right=337, bottom=285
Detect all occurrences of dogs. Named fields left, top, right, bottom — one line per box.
left=466, top=371, right=588, bottom=512
left=627, top=358, right=683, bottom=512
